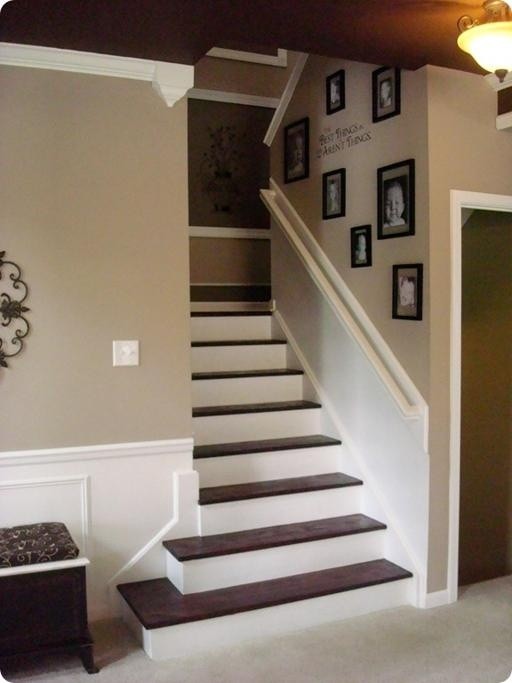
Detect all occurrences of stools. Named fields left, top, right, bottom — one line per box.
left=0, top=522, right=100, bottom=674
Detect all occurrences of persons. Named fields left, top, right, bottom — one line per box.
left=328, top=179, right=338, bottom=212
left=288, top=134, right=304, bottom=175
left=397, top=276, right=417, bottom=307
left=380, top=78, right=392, bottom=108
left=354, top=235, right=367, bottom=265
left=329, top=79, right=340, bottom=103
left=383, top=181, right=405, bottom=227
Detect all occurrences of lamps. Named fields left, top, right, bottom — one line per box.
left=457, top=0, right=512, bottom=84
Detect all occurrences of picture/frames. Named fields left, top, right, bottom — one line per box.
left=377, top=159, right=415, bottom=239
left=322, top=167, right=346, bottom=219
left=392, top=264, right=423, bottom=321
left=283, top=115, right=310, bottom=184
left=326, top=68, right=346, bottom=115
left=205, top=46, right=289, bottom=69
left=372, top=60, right=401, bottom=124
left=350, top=224, right=372, bottom=268
left=188, top=87, right=281, bottom=240
